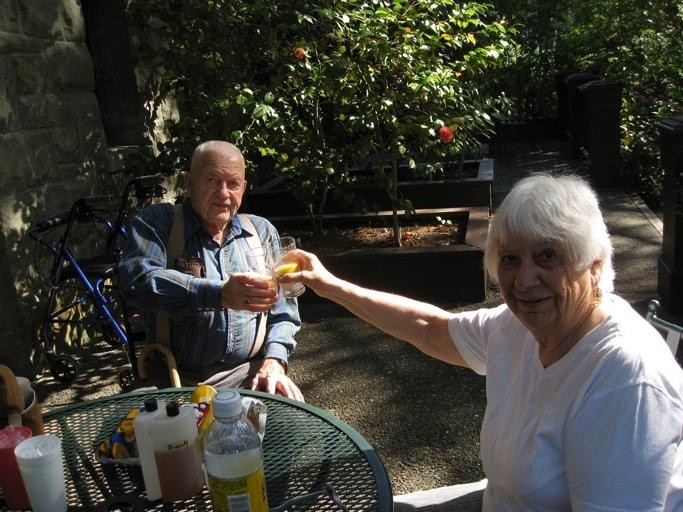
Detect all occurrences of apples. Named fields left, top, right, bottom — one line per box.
left=439, top=125, right=454, bottom=144
left=278, top=154, right=288, bottom=163
left=263, top=92, right=274, bottom=102
left=293, top=45, right=308, bottom=61
left=338, top=113, right=345, bottom=121
left=338, top=45, right=345, bottom=53
left=328, top=168, right=336, bottom=176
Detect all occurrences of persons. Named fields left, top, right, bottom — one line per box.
left=275, top=170, right=683, bottom=512
left=116, top=138, right=303, bottom=403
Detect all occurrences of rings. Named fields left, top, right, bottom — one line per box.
left=244, top=296, right=250, bottom=306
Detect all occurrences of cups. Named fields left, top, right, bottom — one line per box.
left=239, top=248, right=279, bottom=307
left=13, top=434, right=68, bottom=511
left=266, top=237, right=305, bottom=298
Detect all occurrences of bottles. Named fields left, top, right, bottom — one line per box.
left=198, top=390, right=269, bottom=512
left=126, top=397, right=179, bottom=506
left=144, top=400, right=201, bottom=503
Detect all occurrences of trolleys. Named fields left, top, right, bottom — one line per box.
left=20, top=151, right=168, bottom=378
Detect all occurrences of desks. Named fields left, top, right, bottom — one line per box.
left=34, top=386, right=392, bottom=511
